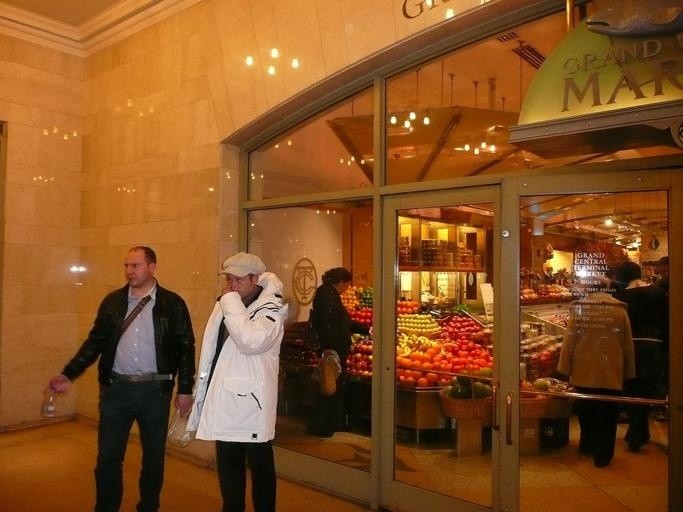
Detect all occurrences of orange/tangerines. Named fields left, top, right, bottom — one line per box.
left=360, top=287, right=373, bottom=308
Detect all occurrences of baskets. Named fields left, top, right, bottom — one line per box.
left=439, top=372, right=494, bottom=421
left=520, top=393, right=572, bottom=419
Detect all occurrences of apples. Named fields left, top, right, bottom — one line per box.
left=438, top=316, right=493, bottom=372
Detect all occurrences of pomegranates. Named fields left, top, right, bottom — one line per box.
left=349, top=308, right=373, bottom=324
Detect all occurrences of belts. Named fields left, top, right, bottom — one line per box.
left=111, top=371, right=172, bottom=383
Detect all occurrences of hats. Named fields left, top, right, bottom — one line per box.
left=217, top=252, right=266, bottom=278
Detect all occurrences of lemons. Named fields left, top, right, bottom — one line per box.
left=397, top=314, right=442, bottom=334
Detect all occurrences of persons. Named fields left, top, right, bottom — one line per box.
left=307, top=267, right=373, bottom=438
left=184, top=250, right=291, bottom=511
left=48, top=247, right=196, bottom=512
left=612, top=261, right=669, bottom=453
left=585, top=254, right=669, bottom=421
left=555, top=276, right=635, bottom=468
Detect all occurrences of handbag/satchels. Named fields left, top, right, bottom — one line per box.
left=319, top=349, right=341, bottom=396
left=304, top=323, right=320, bottom=352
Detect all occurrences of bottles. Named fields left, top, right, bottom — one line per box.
left=40, top=386, right=57, bottom=418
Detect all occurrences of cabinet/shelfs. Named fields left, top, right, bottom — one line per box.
left=398, top=212, right=491, bottom=318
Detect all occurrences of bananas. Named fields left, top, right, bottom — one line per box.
left=396, top=332, right=436, bottom=357
